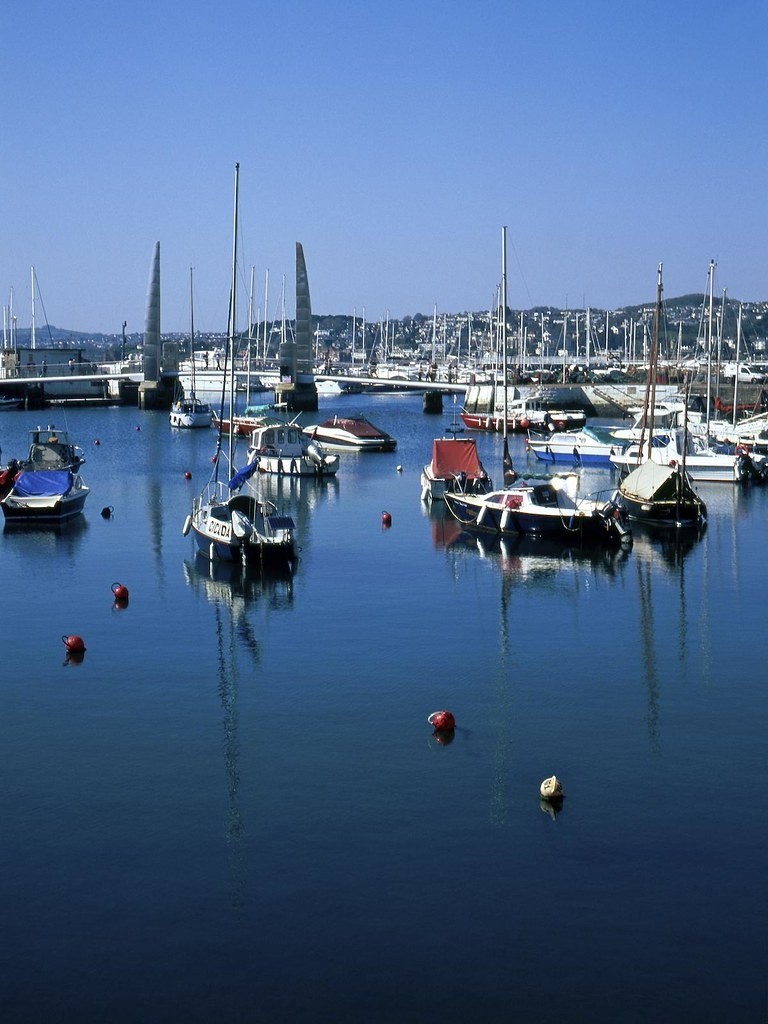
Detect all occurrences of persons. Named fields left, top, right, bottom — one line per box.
left=15, top=360, right=21, bottom=378
left=453, top=364, right=458, bottom=382
left=203, top=351, right=209, bottom=370
left=324, top=357, right=334, bottom=375
left=418, top=359, right=439, bottom=382
left=39, top=360, right=47, bottom=377
left=370, top=359, right=379, bottom=379
left=215, top=354, right=222, bottom=370
left=69, top=358, right=75, bottom=375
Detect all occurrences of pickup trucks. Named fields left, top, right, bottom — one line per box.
left=724, top=364, right=764, bottom=384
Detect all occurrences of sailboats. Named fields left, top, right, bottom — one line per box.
left=609, top=259, right=768, bottom=481
left=214, top=265, right=302, bottom=437
left=443, top=226, right=627, bottom=544
left=611, top=262, right=707, bottom=529
left=0, top=266, right=109, bottom=400
left=179, top=283, right=768, bottom=442
left=191, top=162, right=296, bottom=566
left=169, top=267, right=213, bottom=426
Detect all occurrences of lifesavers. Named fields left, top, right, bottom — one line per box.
left=260, top=445, right=277, bottom=456
left=734, top=444, right=749, bottom=456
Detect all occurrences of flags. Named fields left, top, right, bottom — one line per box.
left=229, top=456, right=260, bottom=490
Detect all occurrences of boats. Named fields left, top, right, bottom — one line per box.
left=527, top=428, right=633, bottom=463
left=302, top=413, right=397, bottom=452
left=0, top=424, right=90, bottom=522
left=459, top=396, right=587, bottom=433
left=421, top=394, right=493, bottom=500
left=247, top=410, right=340, bottom=474
